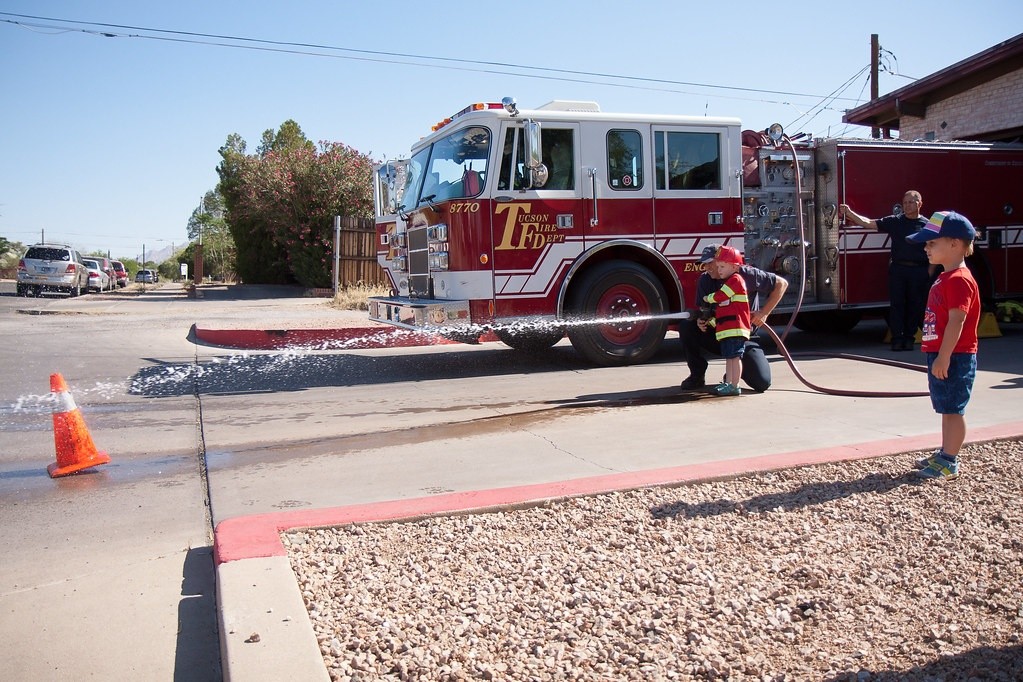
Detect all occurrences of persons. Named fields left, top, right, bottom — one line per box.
left=703, top=246, right=751, bottom=396
left=681, top=243, right=789, bottom=392
left=840, top=190, right=936, bottom=352
left=904, top=210, right=981, bottom=480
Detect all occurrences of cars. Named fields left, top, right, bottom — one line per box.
left=135, top=270, right=160, bottom=284
left=81, top=259, right=110, bottom=293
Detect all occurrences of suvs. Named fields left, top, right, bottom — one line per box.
left=110, top=259, right=129, bottom=289
left=16, top=242, right=90, bottom=298
left=83, top=255, right=120, bottom=291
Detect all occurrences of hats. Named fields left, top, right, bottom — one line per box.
left=713, top=245, right=743, bottom=264
left=904, top=211, right=975, bottom=245
left=695, top=245, right=718, bottom=265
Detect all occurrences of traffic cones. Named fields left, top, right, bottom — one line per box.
left=46, top=370, right=111, bottom=478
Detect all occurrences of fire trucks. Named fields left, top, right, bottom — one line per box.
left=365, top=95, right=1023, bottom=370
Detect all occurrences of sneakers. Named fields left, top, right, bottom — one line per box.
left=916, top=455, right=959, bottom=480
left=915, top=449, right=944, bottom=468
left=717, top=384, right=742, bottom=396
left=713, top=382, right=729, bottom=391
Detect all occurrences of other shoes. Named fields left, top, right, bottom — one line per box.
left=891, top=334, right=915, bottom=351
left=681, top=374, right=705, bottom=389
